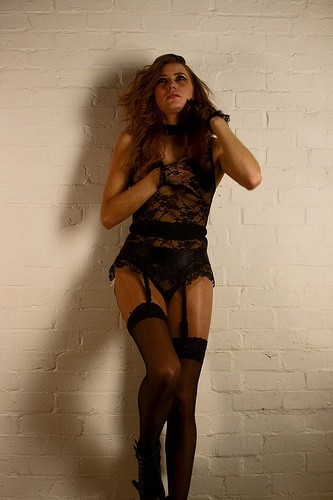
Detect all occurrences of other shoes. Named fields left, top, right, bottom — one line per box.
left=165, top=496, right=170, bottom=500
left=132, top=440, right=165, bottom=500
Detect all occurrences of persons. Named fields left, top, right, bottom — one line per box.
left=101, top=54, right=262, bottom=500
